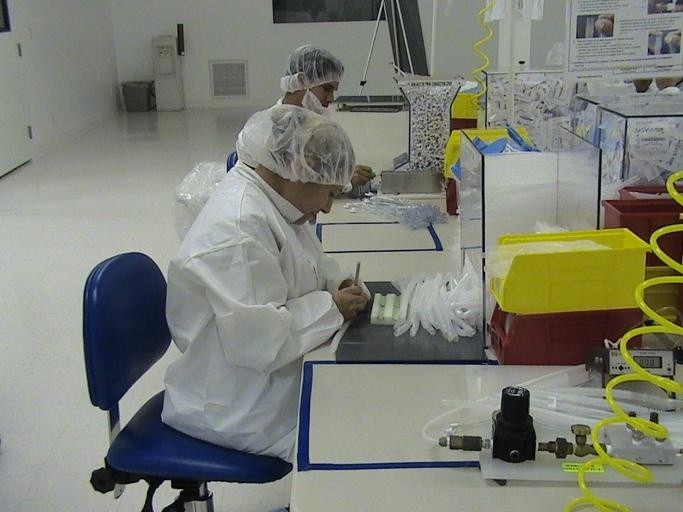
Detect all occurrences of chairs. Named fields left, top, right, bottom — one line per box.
left=227, top=150, right=238, bottom=172
left=80, top=249, right=293, bottom=512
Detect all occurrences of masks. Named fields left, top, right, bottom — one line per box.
left=298, top=86, right=334, bottom=119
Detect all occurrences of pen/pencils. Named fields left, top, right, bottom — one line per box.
left=354, top=263, right=360, bottom=286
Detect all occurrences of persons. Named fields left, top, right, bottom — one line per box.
left=266, top=44, right=376, bottom=199
left=159, top=103, right=372, bottom=509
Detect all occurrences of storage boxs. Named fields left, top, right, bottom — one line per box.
left=484, top=183, right=683, bottom=366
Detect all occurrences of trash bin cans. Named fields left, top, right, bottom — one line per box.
left=121, top=81, right=151, bottom=113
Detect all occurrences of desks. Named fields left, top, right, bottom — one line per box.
left=289, top=95, right=682, bottom=512
